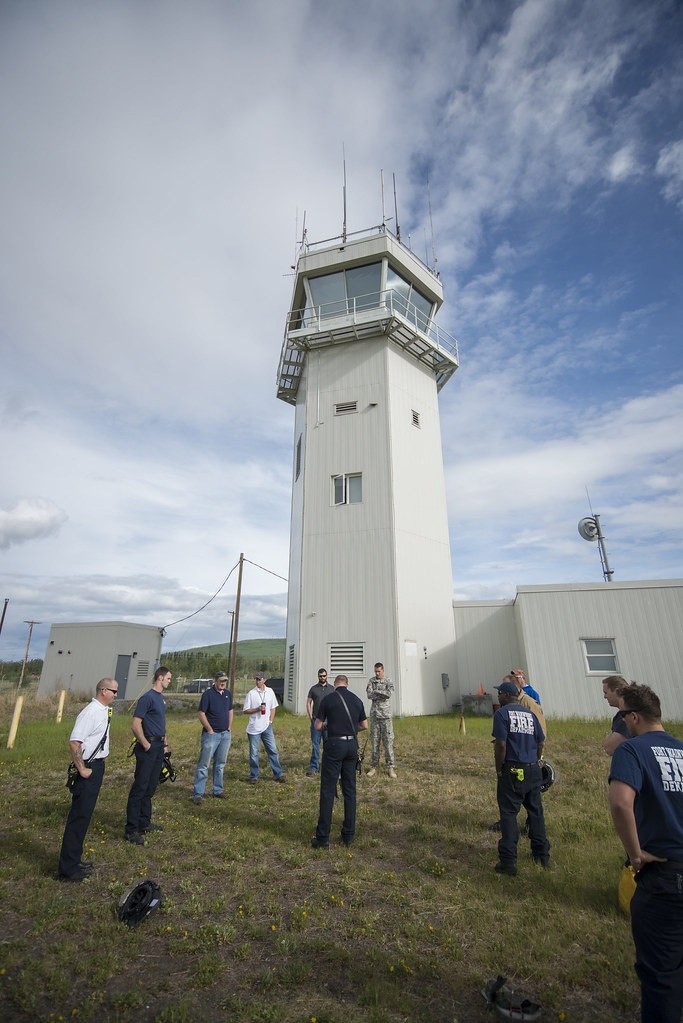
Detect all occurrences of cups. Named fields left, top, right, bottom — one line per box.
left=261, top=703, right=266, bottom=715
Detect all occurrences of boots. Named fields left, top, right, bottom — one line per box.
left=389, top=768, right=397, bottom=779
left=367, top=766, right=376, bottom=777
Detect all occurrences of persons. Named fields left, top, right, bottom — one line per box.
left=602, top=676, right=638, bottom=867
left=491, top=682, right=551, bottom=876
left=366, top=662, right=397, bottom=778
left=511, top=669, right=540, bottom=705
left=193, top=670, right=234, bottom=804
left=243, top=671, right=286, bottom=784
left=489, top=674, right=546, bottom=833
left=311, top=675, right=368, bottom=848
left=608, top=681, right=683, bottom=1023
left=306, top=668, right=334, bottom=775
left=124, top=667, right=172, bottom=846
left=58, top=678, right=119, bottom=883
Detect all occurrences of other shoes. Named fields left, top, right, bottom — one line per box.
left=311, top=837, right=329, bottom=849
left=79, top=861, right=93, bottom=870
left=124, top=771, right=314, bottom=845
left=80, top=870, right=91, bottom=880
left=490, top=821, right=502, bottom=833
left=480, top=974, right=541, bottom=1021
left=495, top=862, right=516, bottom=876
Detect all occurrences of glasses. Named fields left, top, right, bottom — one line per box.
left=619, top=709, right=640, bottom=717
left=102, top=688, right=118, bottom=694
left=319, top=675, right=326, bottom=678
left=498, top=689, right=507, bottom=695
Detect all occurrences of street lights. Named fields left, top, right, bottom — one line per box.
left=226, top=610, right=235, bottom=690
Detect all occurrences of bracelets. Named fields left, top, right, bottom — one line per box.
left=164, top=745, right=168, bottom=747
left=270, top=721, right=273, bottom=724
left=228, top=730, right=230, bottom=732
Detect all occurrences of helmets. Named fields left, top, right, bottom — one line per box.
left=119, top=876, right=161, bottom=928
left=158, top=755, right=173, bottom=784
left=540, top=757, right=558, bottom=793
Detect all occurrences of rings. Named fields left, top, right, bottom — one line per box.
left=636, top=870, right=639, bottom=873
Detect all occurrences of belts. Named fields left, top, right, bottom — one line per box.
left=146, top=736, right=165, bottom=741
left=327, top=735, right=354, bottom=740
left=83, top=757, right=104, bottom=763
left=510, top=762, right=537, bottom=767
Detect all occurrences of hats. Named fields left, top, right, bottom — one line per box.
left=215, top=671, right=228, bottom=681
left=493, top=682, right=520, bottom=697
left=511, top=669, right=526, bottom=679
left=256, top=672, right=266, bottom=679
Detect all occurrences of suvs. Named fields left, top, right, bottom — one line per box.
left=183, top=679, right=216, bottom=694
left=264, top=676, right=285, bottom=704
